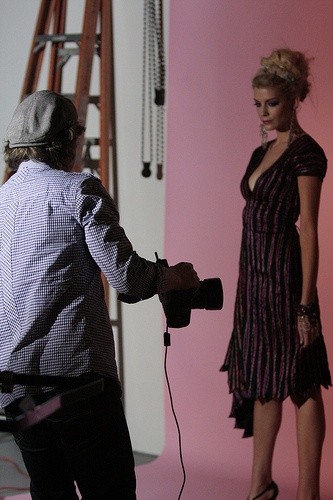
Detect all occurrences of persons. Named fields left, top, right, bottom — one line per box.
left=1, top=90, right=200, bottom=499
left=218, top=47, right=332, bottom=499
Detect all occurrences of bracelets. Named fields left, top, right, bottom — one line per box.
left=296, top=305, right=314, bottom=317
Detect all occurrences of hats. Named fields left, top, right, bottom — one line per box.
left=5, top=90, right=78, bottom=148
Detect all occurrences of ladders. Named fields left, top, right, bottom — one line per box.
left=0, top=0, right=109, bottom=321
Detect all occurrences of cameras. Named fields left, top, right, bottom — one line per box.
left=166, top=277, right=224, bottom=329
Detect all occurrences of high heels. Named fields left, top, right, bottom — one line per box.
left=247, top=482, right=278, bottom=500
